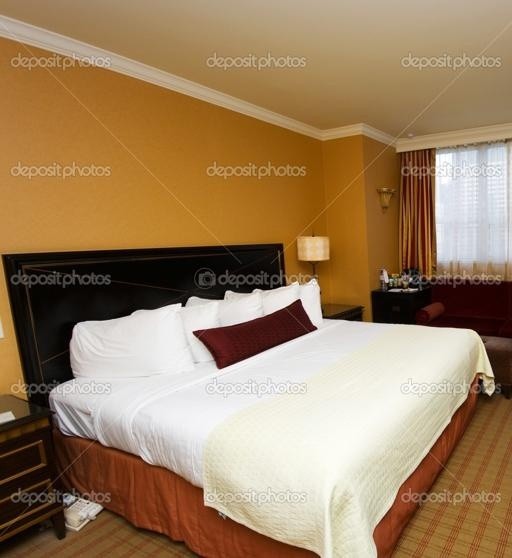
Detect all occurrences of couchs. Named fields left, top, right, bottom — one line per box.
left=417, top=280, right=512, bottom=338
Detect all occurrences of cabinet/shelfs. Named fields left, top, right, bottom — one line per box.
left=371, top=286, right=432, bottom=325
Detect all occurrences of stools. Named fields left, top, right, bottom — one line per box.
left=480, top=335, right=512, bottom=399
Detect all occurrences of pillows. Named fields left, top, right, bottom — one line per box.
left=415, top=302, right=445, bottom=324
left=69, top=278, right=323, bottom=378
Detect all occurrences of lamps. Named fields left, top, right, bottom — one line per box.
left=296, top=230, right=330, bottom=312
left=377, top=185, right=397, bottom=213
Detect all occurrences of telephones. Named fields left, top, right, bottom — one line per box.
left=63, top=493, right=104, bottom=532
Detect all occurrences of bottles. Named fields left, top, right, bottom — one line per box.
left=380, top=270, right=388, bottom=292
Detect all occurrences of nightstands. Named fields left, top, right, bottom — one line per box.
left=322, top=304, right=365, bottom=322
left=0, top=394, right=67, bottom=543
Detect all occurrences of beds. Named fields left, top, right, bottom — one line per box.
left=1, top=243, right=479, bottom=558
